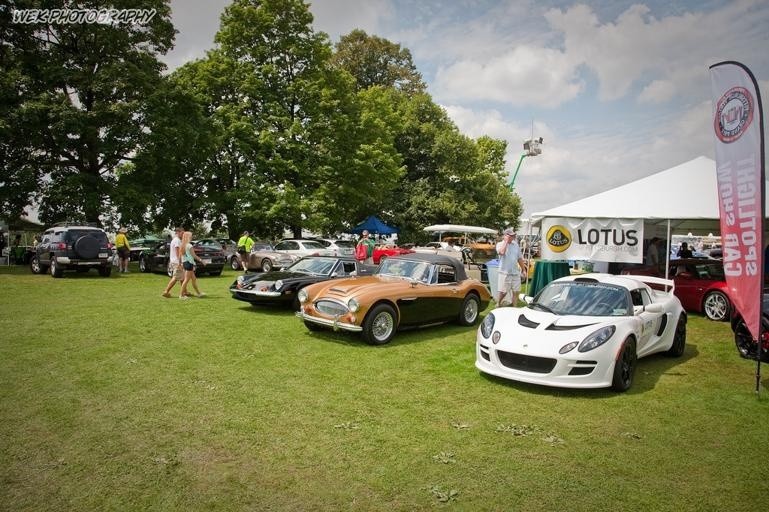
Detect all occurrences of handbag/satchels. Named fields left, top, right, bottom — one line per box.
left=183, top=261, right=193, bottom=271
left=118, top=247, right=131, bottom=256
left=238, top=246, right=245, bottom=254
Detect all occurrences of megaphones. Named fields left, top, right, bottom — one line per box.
left=533, top=137, right=545, bottom=144
left=522, top=137, right=533, bottom=144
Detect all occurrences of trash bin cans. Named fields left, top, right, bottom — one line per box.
left=480, top=263, right=489, bottom=283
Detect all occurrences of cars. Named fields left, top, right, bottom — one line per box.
left=666, top=241, right=723, bottom=265
left=620, top=259, right=741, bottom=323
left=729, top=284, right=768, bottom=360
left=397, top=234, right=498, bottom=261
left=1, top=222, right=358, bottom=280
left=519, top=251, right=536, bottom=284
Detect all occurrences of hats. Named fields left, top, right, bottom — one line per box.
left=119, top=228, right=127, bottom=233
left=504, top=229, right=516, bottom=235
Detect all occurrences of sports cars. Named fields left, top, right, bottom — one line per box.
left=229, top=253, right=362, bottom=311
left=475, top=272, right=690, bottom=393
left=294, top=252, right=494, bottom=346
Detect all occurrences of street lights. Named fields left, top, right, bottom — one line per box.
left=509, top=134, right=546, bottom=193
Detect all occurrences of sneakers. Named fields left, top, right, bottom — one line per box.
left=162, top=293, right=171, bottom=298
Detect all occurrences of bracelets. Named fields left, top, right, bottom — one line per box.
left=199, top=259, right=202, bottom=262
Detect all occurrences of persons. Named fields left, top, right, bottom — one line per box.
left=445, top=238, right=459, bottom=252
left=36, top=235, right=42, bottom=242
left=494, top=229, right=527, bottom=308
left=107, top=227, right=118, bottom=266
left=116, top=227, right=132, bottom=272
left=356, top=229, right=373, bottom=267
left=645, top=237, right=660, bottom=274
left=178, top=231, right=207, bottom=300
left=676, top=241, right=694, bottom=258
left=236, top=230, right=255, bottom=274
left=32, top=236, right=38, bottom=247
left=12, top=234, right=22, bottom=246
left=162, top=227, right=191, bottom=297
